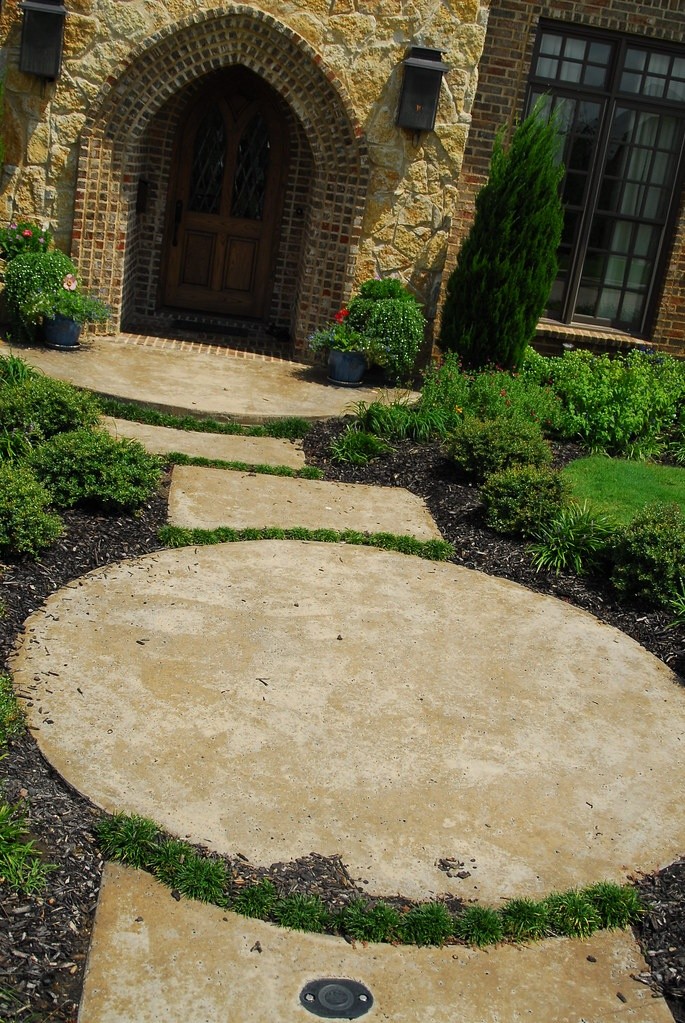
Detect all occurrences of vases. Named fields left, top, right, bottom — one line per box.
left=326, top=347, right=365, bottom=387
left=44, top=312, right=82, bottom=351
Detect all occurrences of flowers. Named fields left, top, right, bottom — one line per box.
left=301, top=275, right=428, bottom=375
left=0, top=219, right=114, bottom=326
